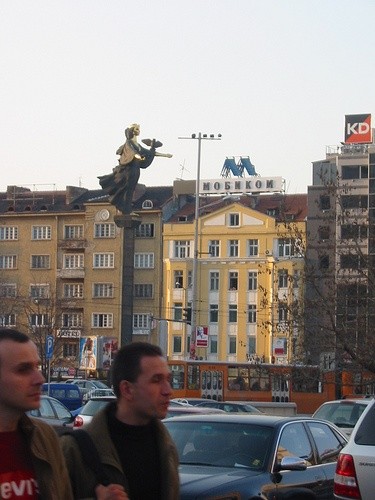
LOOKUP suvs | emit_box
[333,397,375,500]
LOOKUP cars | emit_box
[160,414,351,500]
[308,396,372,444]
[25,378,268,436]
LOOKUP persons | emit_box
[116,124,173,190]
[62,341,180,500]
[0,329,75,500]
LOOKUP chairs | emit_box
[231,434,267,467]
[183,436,222,465]
